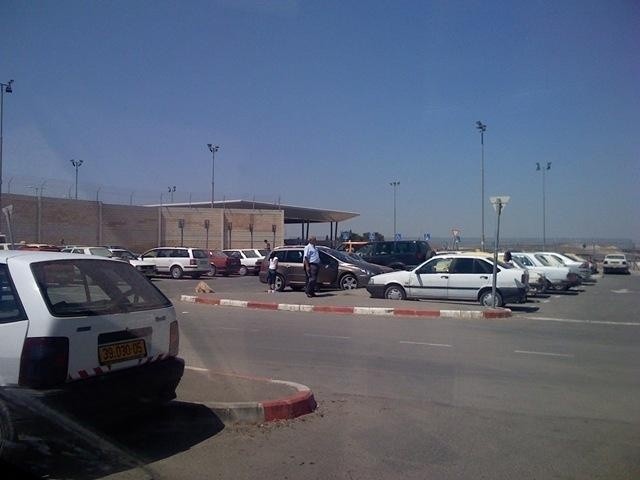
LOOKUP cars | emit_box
[602,251,629,275]
[0,236,268,283]
[366,249,599,309]
[0,253,183,459]
[342,251,363,261]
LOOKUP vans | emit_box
[349,240,436,273]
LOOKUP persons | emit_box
[303,236,320,298]
[266,251,279,293]
[503,251,512,262]
[263,239,270,249]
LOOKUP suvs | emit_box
[333,240,369,255]
[258,244,385,294]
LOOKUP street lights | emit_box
[474,117,490,248]
[389,180,401,242]
[69,157,86,201]
[206,142,222,210]
[534,158,553,251]
[1,77,14,232]
[167,183,178,209]
